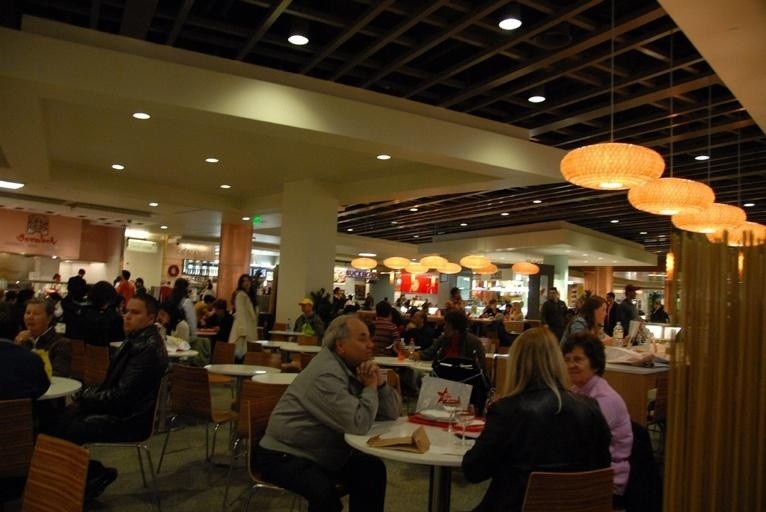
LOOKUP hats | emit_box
[298,297,314,306]
[173,277,189,290]
[625,284,640,293]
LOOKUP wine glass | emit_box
[442,394,475,448]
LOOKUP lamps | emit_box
[350,207,541,277]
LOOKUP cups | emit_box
[669,342,687,363]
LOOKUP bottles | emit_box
[636,333,666,354]
[286,318,292,332]
[613,321,624,348]
[187,260,217,276]
[398,338,414,360]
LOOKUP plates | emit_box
[419,408,451,421]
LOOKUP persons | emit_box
[364,293,434,351]
[255,315,401,512]
[445,287,466,315]
[295,287,356,336]
[160,275,258,362]
[541,285,667,347]
[480,299,523,320]
[462,328,632,511]
[410,312,487,376]
[1,269,169,502]
